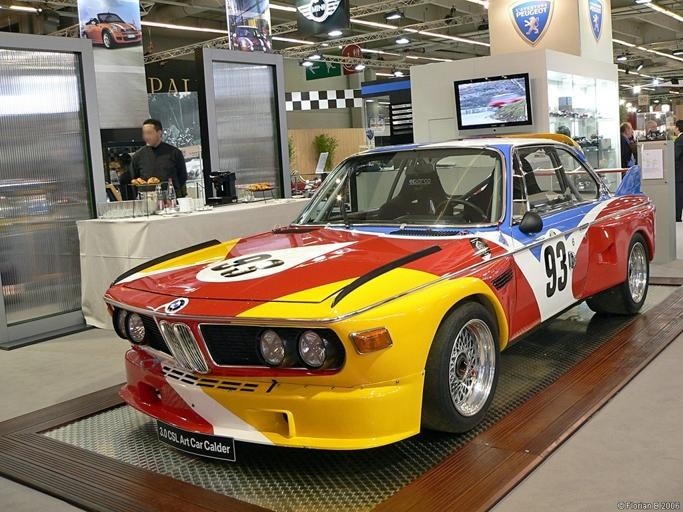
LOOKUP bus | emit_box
[0,177,54,220]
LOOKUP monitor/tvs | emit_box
[453,72,534,136]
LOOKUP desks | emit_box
[76,197,314,330]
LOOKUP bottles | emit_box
[166,177,177,209]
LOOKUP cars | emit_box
[487,92,523,111]
[95,128,657,466]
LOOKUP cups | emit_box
[96,199,148,219]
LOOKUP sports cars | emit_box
[230,24,270,52]
[80,9,141,50]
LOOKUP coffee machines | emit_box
[204,170,238,204]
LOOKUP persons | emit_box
[640,121,658,141]
[619,121,638,180]
[674,118,683,224]
[127,117,188,201]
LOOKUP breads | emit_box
[131,177,161,184]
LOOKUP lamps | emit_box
[302,9,412,78]
[618,51,662,112]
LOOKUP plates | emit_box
[127,183,163,186]
[245,187,275,192]
